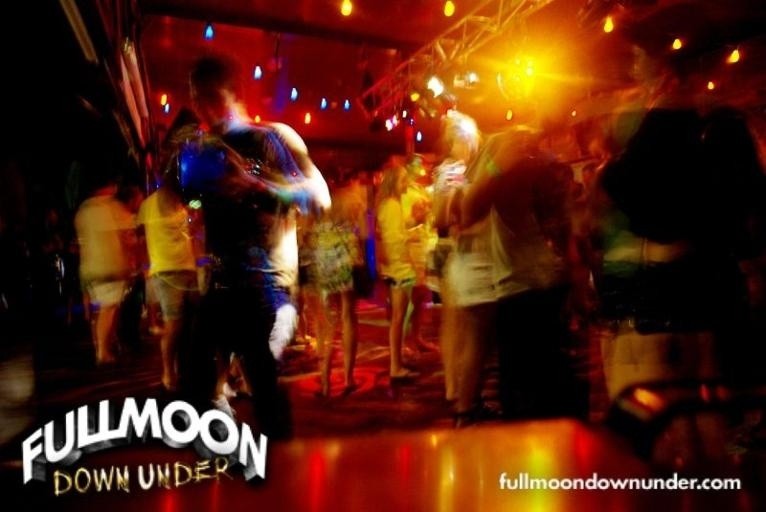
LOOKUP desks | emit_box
[0,416,704,512]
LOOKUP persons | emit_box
[76,9,766,512]
